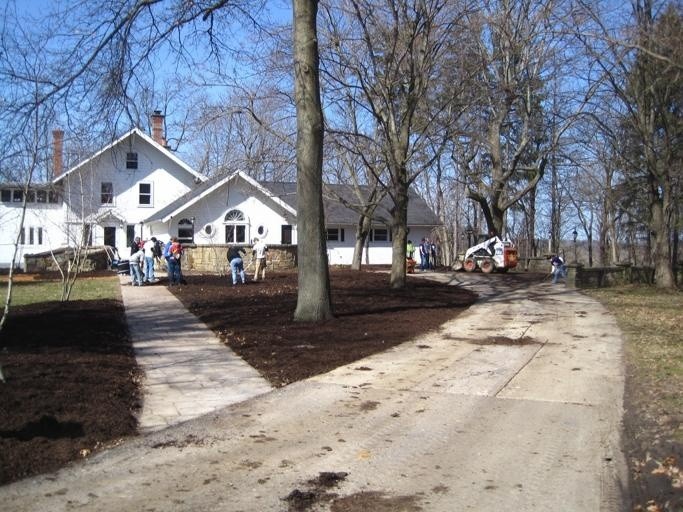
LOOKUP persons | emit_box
[557,247,567,276]
[406,237,436,272]
[250,237,269,283]
[225,243,248,287]
[546,254,567,284]
[127,235,184,287]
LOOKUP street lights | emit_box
[571,230,579,263]
[460,222,477,248]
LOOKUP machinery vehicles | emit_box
[451,232,519,273]
